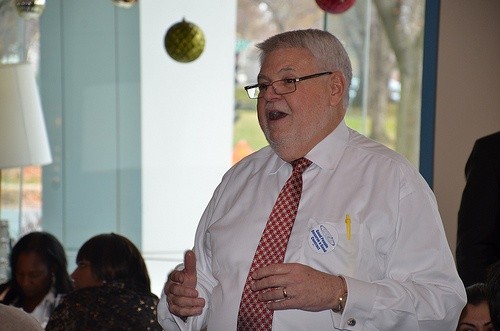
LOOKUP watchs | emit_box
[337,288,349,313]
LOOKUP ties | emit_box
[236,157,315,331]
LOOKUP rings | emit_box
[283,286,287,298]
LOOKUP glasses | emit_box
[244,71,333,98]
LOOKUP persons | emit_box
[0,230,74,331]
[456,283,495,331]
[156,28,469,331]
[454,131,500,331]
[44,232,166,331]
